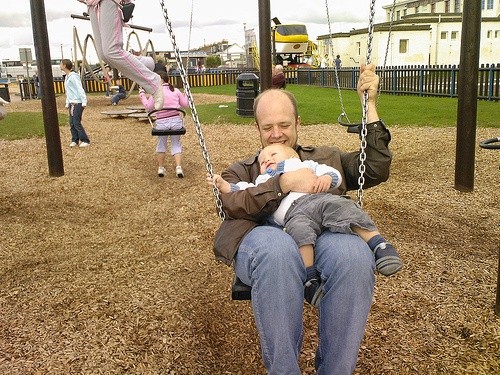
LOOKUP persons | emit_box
[60,58,91,148]
[78,0,166,110]
[138,71,191,178]
[206,142,402,308]
[212,63,393,375]
[30,54,342,107]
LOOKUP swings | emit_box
[159,0,376,307]
[325,0,397,133]
[123,0,193,137]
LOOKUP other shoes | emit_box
[373,241,403,276]
[303,277,325,308]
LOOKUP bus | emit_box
[1,59,66,78]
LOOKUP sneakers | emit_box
[175,166,185,178]
[79,142,90,148]
[69,142,77,146]
[157,166,168,177]
[153,75,165,110]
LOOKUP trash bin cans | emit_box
[236,73,259,116]
[0,83,10,102]
